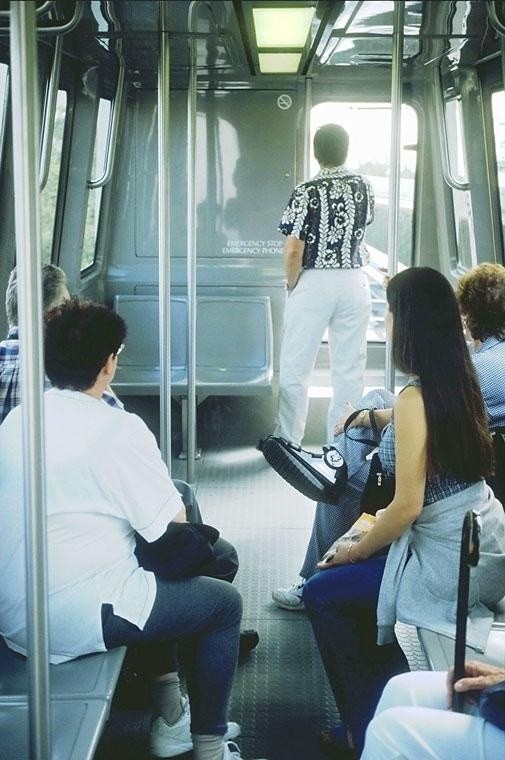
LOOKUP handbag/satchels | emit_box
[360,453,388,516]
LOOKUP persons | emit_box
[303,265,505,760]
[2,259,259,656]
[255,121,376,455]
[358,655,504,759]
[3,297,247,759]
[268,261,505,613]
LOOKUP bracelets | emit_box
[348,542,358,563]
[285,281,294,291]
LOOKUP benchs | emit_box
[0,480,195,758]
[104,295,274,460]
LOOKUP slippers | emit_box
[321,724,355,754]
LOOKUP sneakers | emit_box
[271,579,309,612]
[237,630,259,657]
[150,697,242,759]
[262,436,349,504]
